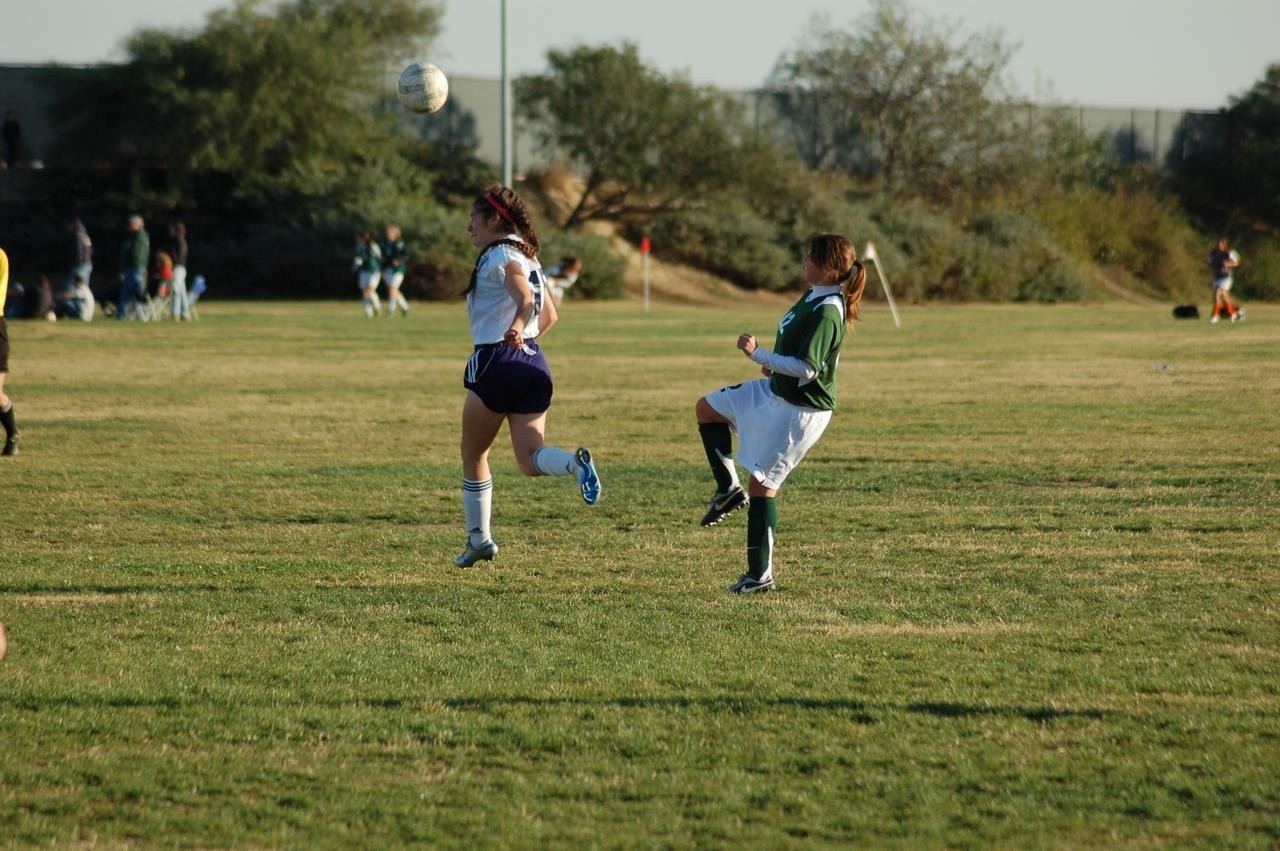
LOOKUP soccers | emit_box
[397,61,448,114]
[45,311,58,323]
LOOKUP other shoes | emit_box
[1209,314,1220,324]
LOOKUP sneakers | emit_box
[2,432,20,456]
[575,447,601,506]
[729,574,776,594]
[455,537,499,569]
[700,484,750,528]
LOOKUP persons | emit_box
[695,230,867,598]
[1206,238,1242,324]
[0,248,22,455]
[544,255,583,310]
[453,183,601,568]
[344,224,410,319]
[5,214,188,318]
